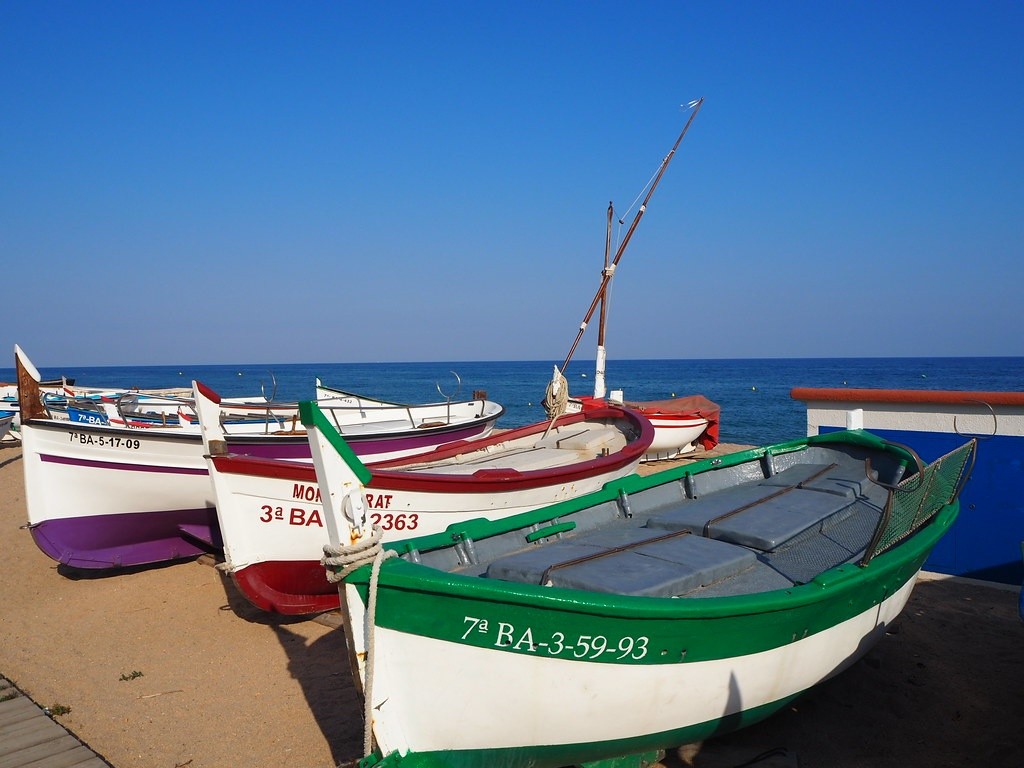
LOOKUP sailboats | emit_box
[545,99,719,465]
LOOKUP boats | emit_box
[192,379,653,619]
[10,343,504,576]
[296,399,976,767]
[1,378,300,449]
